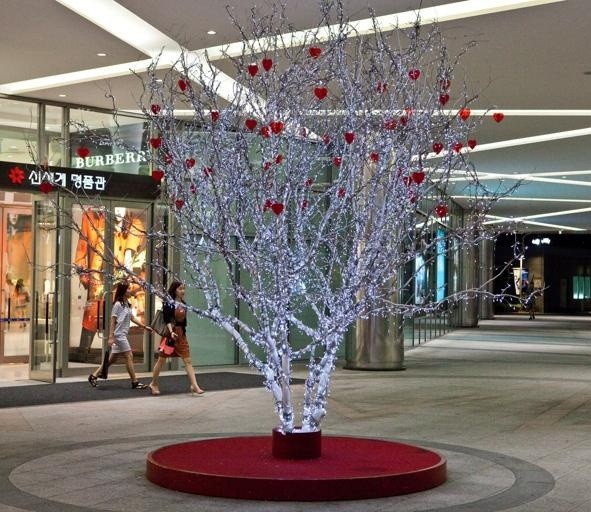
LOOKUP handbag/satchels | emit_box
[150,302,177,337]
[97,345,109,379]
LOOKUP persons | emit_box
[148,282,206,394]
[88,280,154,390]
[524,281,540,320]
[13,279,31,329]
[8,213,25,235]
[2,272,16,317]
[92,203,146,330]
[74,204,143,363]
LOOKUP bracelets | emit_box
[170,330,174,334]
[143,325,149,329]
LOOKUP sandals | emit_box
[131,381,148,389]
[87,374,98,387]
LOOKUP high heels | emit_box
[148,383,160,395]
[190,386,205,396]
